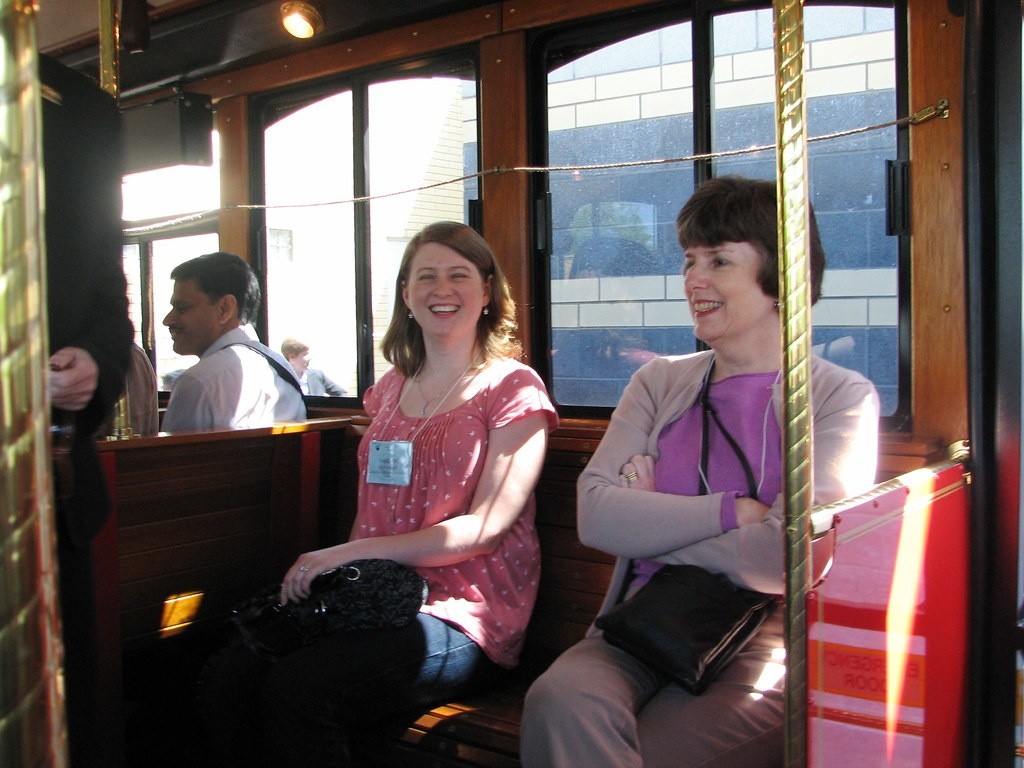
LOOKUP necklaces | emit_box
[418,381,445,415]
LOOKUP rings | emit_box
[626,471,638,484]
[301,567,307,572]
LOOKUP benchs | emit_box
[93,417,348,711]
[343,417,942,768]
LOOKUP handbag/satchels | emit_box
[223,558,429,648]
[594,562,776,693]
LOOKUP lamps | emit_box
[280,0,326,39]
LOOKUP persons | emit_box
[196,224,559,767]
[519,174,880,767]
[161,251,307,433]
[38,53,134,768]
[282,338,347,397]
